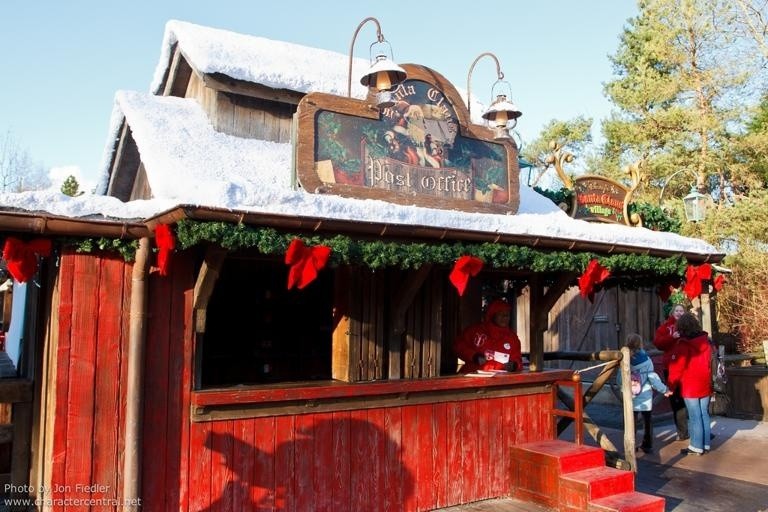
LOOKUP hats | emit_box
[396,101,423,119]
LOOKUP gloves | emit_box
[472,353,486,366]
[505,361,517,372]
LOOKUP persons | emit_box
[610,332,674,454]
[454,300,524,375]
[667,314,714,455]
[651,303,691,440]
[383,104,443,169]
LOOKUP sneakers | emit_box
[688,445,711,453]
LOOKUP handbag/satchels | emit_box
[709,352,731,415]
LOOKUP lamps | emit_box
[345,14,525,142]
[657,167,708,228]
